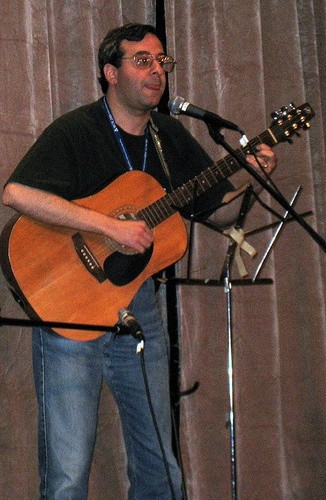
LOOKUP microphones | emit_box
[167,95,239,132]
[118,307,145,343]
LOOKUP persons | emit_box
[0,24,279,500]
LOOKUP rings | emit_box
[264,163,269,168]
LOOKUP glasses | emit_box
[121,53,177,73]
